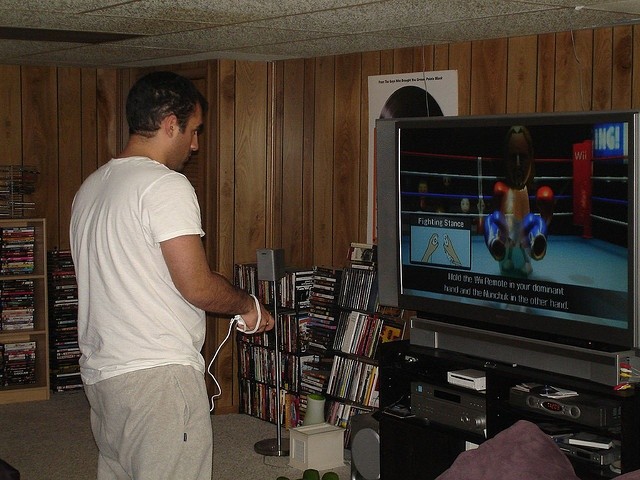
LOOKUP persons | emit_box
[69,69,275,479]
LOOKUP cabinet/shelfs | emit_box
[236,304,309,430]
[375,338,640,479]
[316,259,397,450]
[0,216,50,405]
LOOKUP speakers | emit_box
[352,412,381,479]
[256,248,287,282]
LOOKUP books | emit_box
[324,400,376,449]
[239,308,308,353]
[0,279,35,332]
[238,342,298,395]
[0,226,37,276]
[238,379,297,434]
[326,354,381,410]
[1,342,37,387]
[298,268,336,427]
[331,311,404,359]
[336,267,378,315]
[234,264,313,308]
[48,249,84,391]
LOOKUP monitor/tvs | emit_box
[375,110,639,388]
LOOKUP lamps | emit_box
[253,245,291,457]
